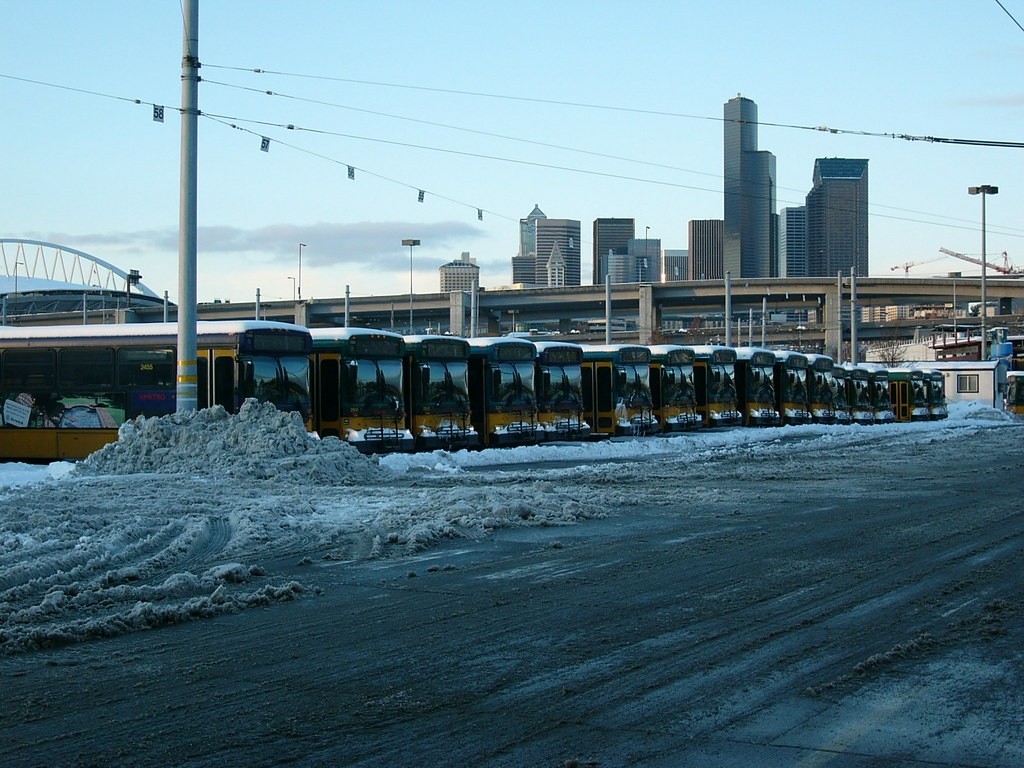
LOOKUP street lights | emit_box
[968,184,999,361]
[645,225,651,258]
[14,262,23,315]
[288,276,295,300]
[401,239,421,335]
[298,242,307,300]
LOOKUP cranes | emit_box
[890,255,947,277]
[939,247,1015,275]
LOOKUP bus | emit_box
[1005,371,1024,414]
[0,319,949,462]
